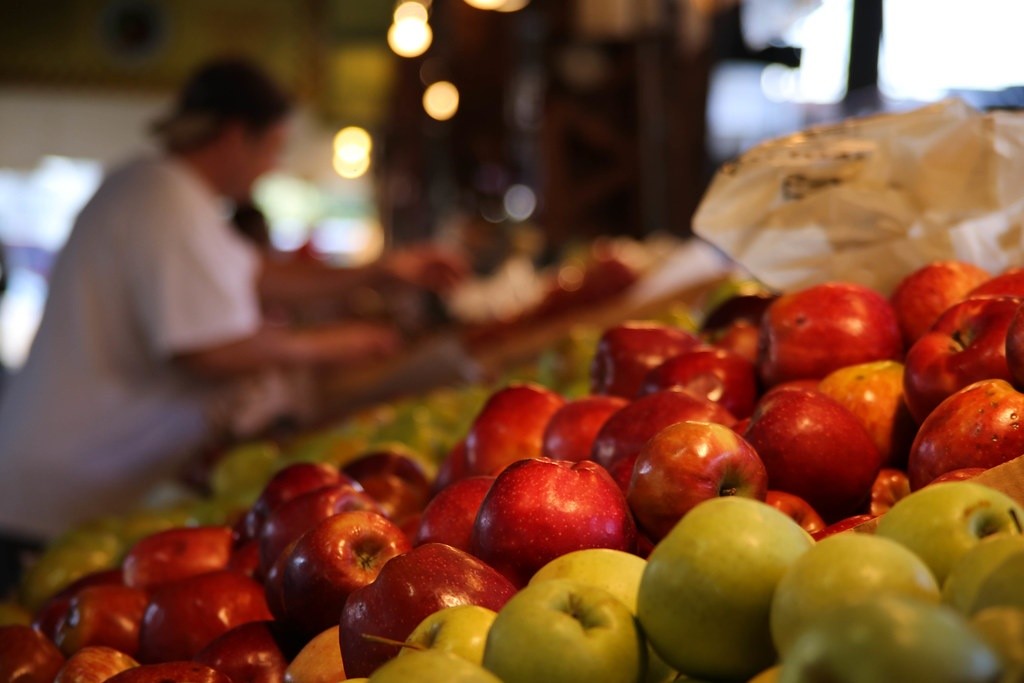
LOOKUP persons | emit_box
[0,51,405,602]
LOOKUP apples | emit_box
[0,259,1024,683]
